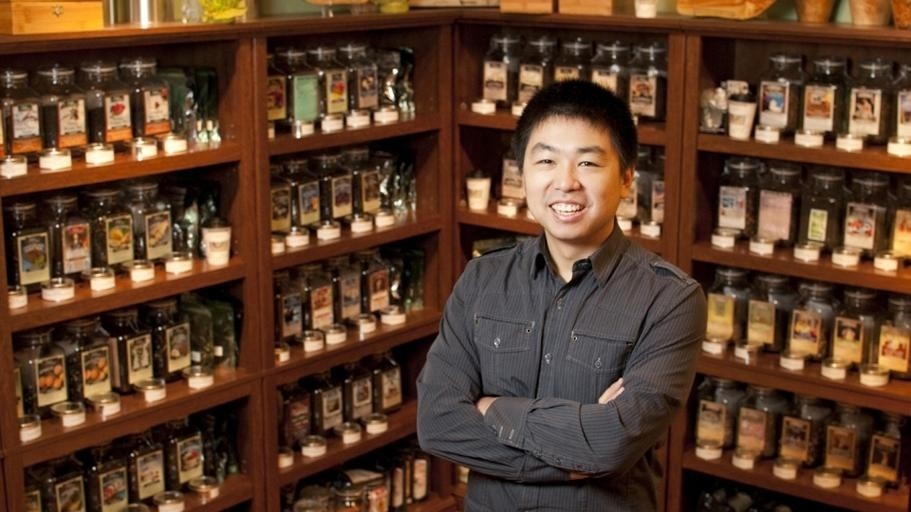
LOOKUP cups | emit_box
[464,169,492,211]
[792,0,911,29]
[632,0,660,19]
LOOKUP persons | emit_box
[417,77,710,511]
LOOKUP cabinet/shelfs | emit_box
[588,39,632,102]
[514,31,559,103]
[0,6,911,512]
[498,131,666,227]
[625,39,666,122]
[477,31,523,108]
[553,37,594,89]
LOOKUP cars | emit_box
[464,169,492,211]
[792,0,911,29]
[632,0,660,19]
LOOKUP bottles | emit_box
[588,39,632,102]
[477,31,523,108]
[625,39,666,122]
[689,48,910,510]
[514,31,559,103]
[498,131,666,227]
[267,32,435,511]
[553,37,594,89]
[0,53,235,510]
